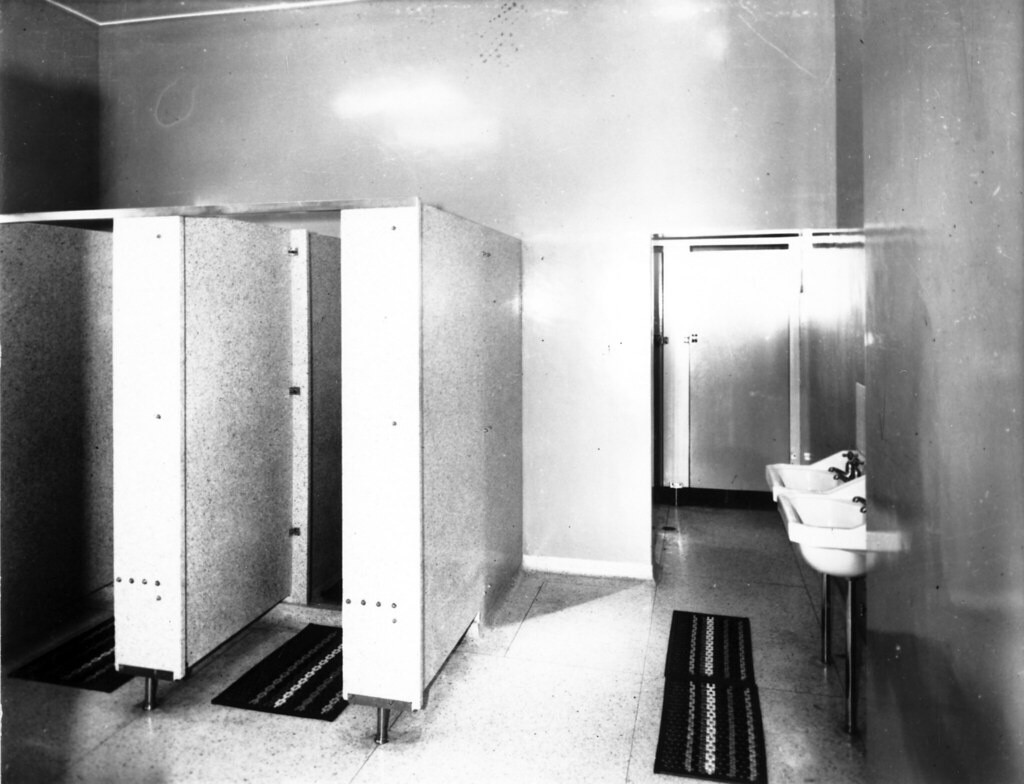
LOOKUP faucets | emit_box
[852,496,867,513]
[829,452,862,481]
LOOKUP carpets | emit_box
[655,610,768,784]
[6,618,135,696]
[208,622,349,724]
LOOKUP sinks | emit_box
[778,475,868,576]
[765,450,871,503]
